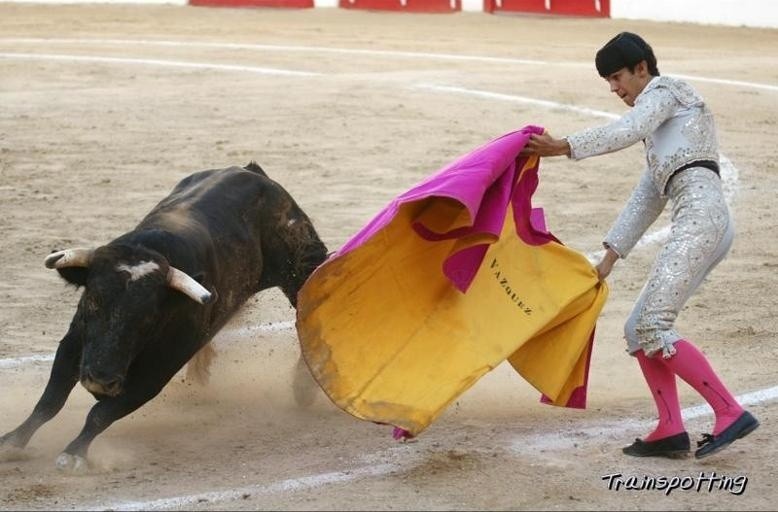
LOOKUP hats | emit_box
[595,32,650,79]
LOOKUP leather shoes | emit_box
[622,429,691,458]
[694,409,761,460]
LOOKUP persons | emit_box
[521,32,760,458]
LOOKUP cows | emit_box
[0,158,334,480]
